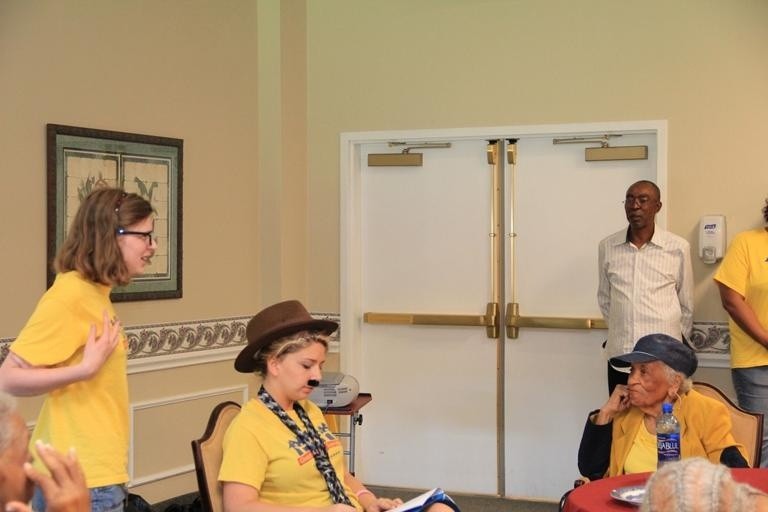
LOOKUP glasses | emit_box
[118,230,153,247]
[623,198,658,206]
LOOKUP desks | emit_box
[321,393,372,477]
[564,468,768,512]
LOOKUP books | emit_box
[385,487,461,512]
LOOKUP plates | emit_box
[610,486,648,506]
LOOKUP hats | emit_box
[235,300,338,373]
[639,457,768,512]
[610,335,697,377]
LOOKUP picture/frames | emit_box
[46,124,183,303]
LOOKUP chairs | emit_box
[693,382,765,468]
[192,402,242,512]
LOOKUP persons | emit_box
[1,188,160,512]
[0,391,91,512]
[597,181,694,402]
[713,198,768,467]
[216,299,456,512]
[578,334,752,482]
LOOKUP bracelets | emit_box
[356,489,374,497]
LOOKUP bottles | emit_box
[655,404,682,470]
